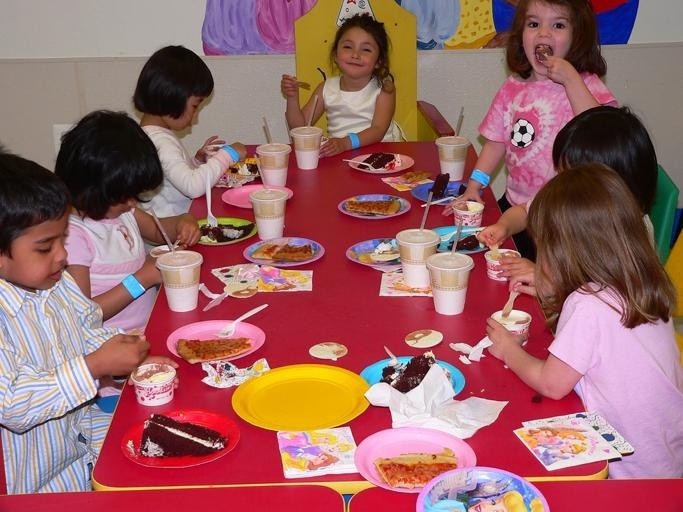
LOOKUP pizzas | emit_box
[251,244,312,263]
[345,199,404,216]
[176,338,252,364]
[374,447,458,489]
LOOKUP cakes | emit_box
[356,152,396,170]
[428,173,450,202]
[457,184,471,199]
[447,235,483,252]
[138,412,226,459]
[199,219,255,240]
[215,155,261,188]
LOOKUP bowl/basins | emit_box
[150,244,178,259]
[321,137,329,158]
[453,201,485,228]
[205,143,225,160]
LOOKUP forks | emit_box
[217,303,271,338]
[206,171,219,227]
[342,158,383,173]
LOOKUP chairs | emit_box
[291,1,455,143]
[647,163,679,266]
[662,231,683,367]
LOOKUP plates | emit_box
[121,411,242,469]
[349,152,414,174]
[222,185,294,208]
[416,467,551,512]
[243,237,325,267]
[360,357,466,410]
[412,181,482,207]
[166,319,266,363]
[231,363,372,433]
[338,194,412,220]
[425,225,496,255]
[187,218,257,246]
[346,237,405,264]
[354,427,477,493]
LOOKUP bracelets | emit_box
[469,169,491,190]
[121,274,146,301]
[223,143,240,164]
[346,132,360,150]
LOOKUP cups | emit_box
[434,136,470,182]
[156,250,203,312]
[250,188,288,239]
[290,126,323,169]
[427,252,475,315]
[255,142,292,187]
[396,228,440,289]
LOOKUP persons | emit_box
[281,10,404,158]
[54,108,202,399]
[131,45,247,251]
[0,142,151,495]
[485,162,683,480]
[440,1,618,217]
[476,107,658,251]
